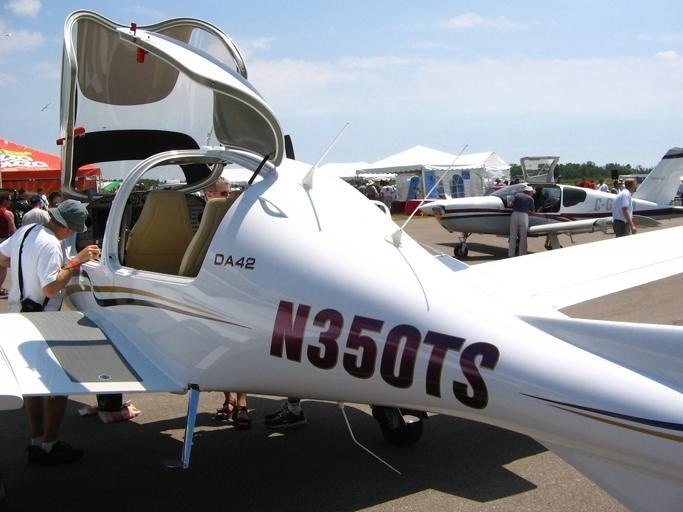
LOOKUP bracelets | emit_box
[70,258,79,271]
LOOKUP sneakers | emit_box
[266,406,307,428]
[28,440,84,466]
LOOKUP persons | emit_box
[493,178,520,191]
[199,176,252,432]
[508,186,537,258]
[0,199,100,467]
[356,178,397,213]
[264,397,307,430]
[0,187,63,297]
[611,179,637,238]
[576,177,626,194]
[679,180,683,206]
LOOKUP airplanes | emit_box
[0,5,682,512]
[413,144,682,260]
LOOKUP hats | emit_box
[48,200,89,233]
[523,186,536,193]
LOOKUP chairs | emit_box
[128,188,196,275]
[174,189,242,277]
[535,196,559,214]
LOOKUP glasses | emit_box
[212,191,228,196]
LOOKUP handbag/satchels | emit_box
[20,299,43,312]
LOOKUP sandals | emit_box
[216,399,251,430]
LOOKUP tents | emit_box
[423,151,511,200]
[0,138,100,195]
